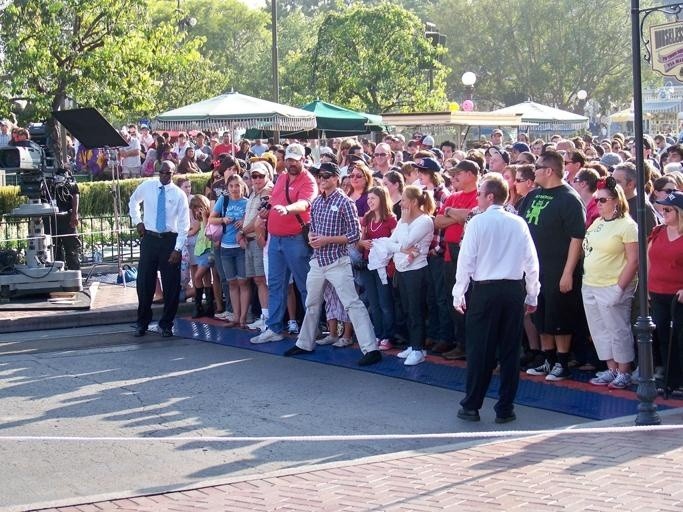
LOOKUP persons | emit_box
[42,175,81,270]
[451,170,544,425]
[66,133,123,181]
[0,120,30,186]
[117,118,683,400]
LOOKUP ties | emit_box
[155,186,166,231]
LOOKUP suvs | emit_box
[28,122,49,153]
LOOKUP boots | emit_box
[191,286,204,318]
[205,286,214,317]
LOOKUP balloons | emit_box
[446,99,476,114]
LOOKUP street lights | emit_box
[417,31,446,93]
[462,70,476,100]
[576,89,586,115]
[664,80,674,94]
[179,14,196,40]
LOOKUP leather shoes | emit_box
[162,326,172,337]
[495,411,516,423]
[457,407,480,421]
[135,326,148,336]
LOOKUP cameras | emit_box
[257,201,272,211]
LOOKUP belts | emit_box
[146,231,175,239]
[246,237,255,241]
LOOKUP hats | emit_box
[320,163,340,174]
[251,163,266,174]
[493,129,502,138]
[347,153,369,166]
[285,144,304,161]
[656,191,683,207]
[411,158,440,171]
[448,160,480,174]
[488,143,536,164]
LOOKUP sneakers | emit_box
[288,320,299,334]
[379,338,467,365]
[315,336,353,347]
[359,351,382,366]
[285,346,312,356]
[590,366,683,399]
[519,349,572,381]
[214,311,284,343]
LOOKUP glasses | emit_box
[595,198,611,203]
[374,153,387,156]
[317,172,331,179]
[350,174,362,178]
[252,175,264,178]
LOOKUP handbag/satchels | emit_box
[302,223,314,249]
[118,264,137,283]
[205,222,224,240]
